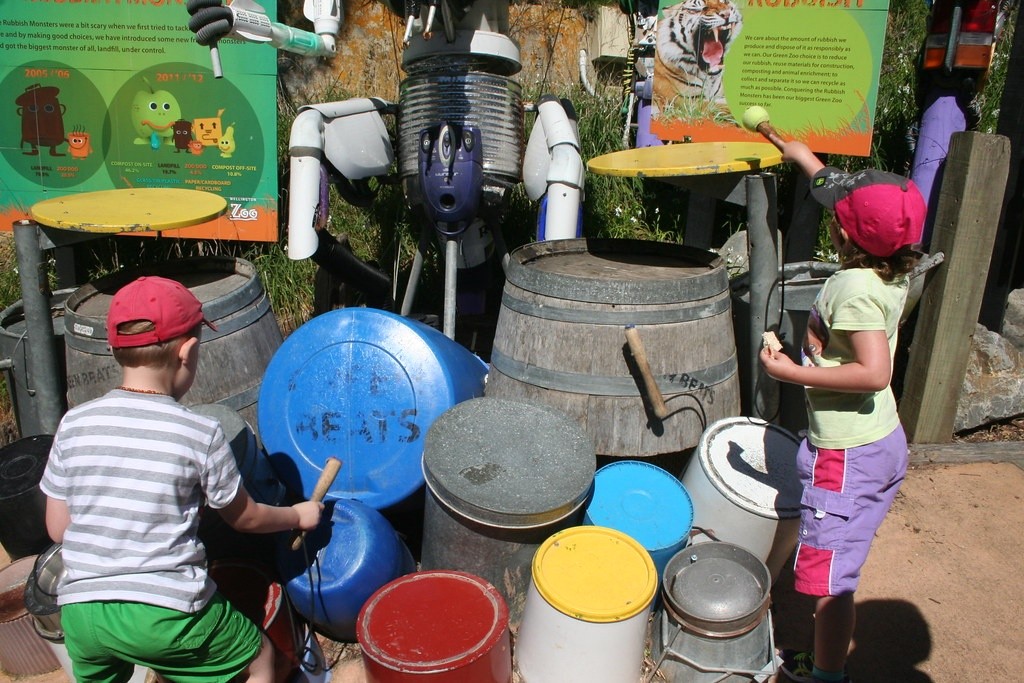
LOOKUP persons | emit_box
[760,140,926,683]
[39,276,325,683]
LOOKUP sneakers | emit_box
[779,649,851,683]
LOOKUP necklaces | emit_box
[115,386,169,395]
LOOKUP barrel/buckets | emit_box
[0,288,84,437]
[730,261,844,437]
[514,525,658,683]
[678,416,802,586]
[63,255,286,452]
[257,306,489,509]
[420,397,595,650]
[0,434,55,561]
[486,239,741,456]
[23,544,151,683]
[581,461,695,613]
[275,499,416,643]
[356,570,513,683]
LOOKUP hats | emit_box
[810,165,928,259]
[107,275,218,349]
